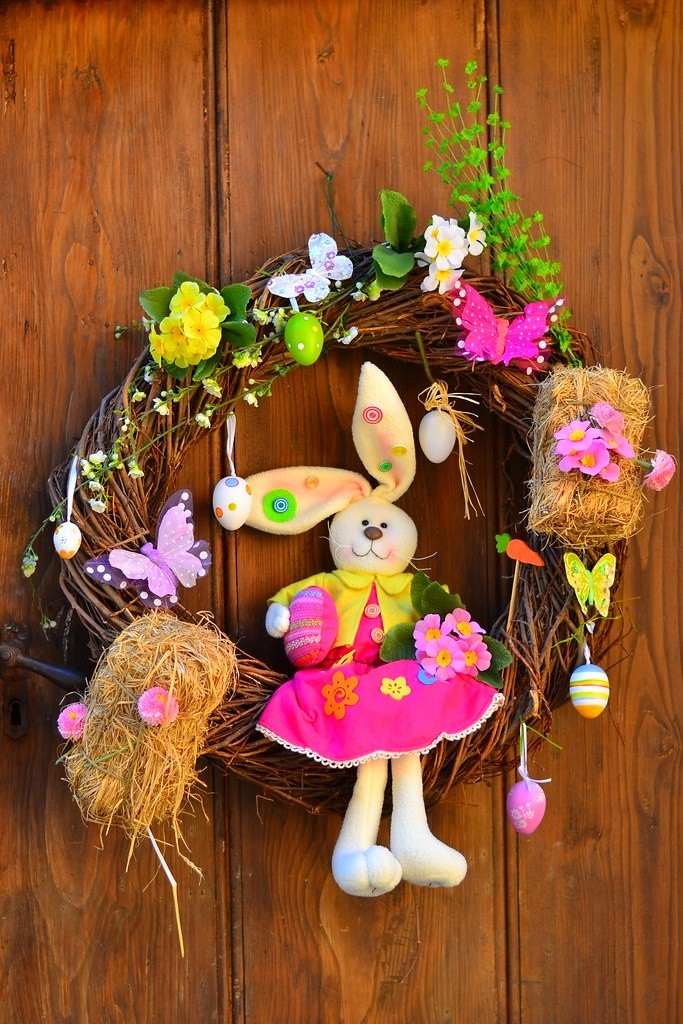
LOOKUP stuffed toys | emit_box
[240,362,505,898]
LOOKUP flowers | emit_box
[137,688,181,728]
[553,401,676,492]
[19,53,587,638]
[56,702,89,740]
[378,570,513,689]
